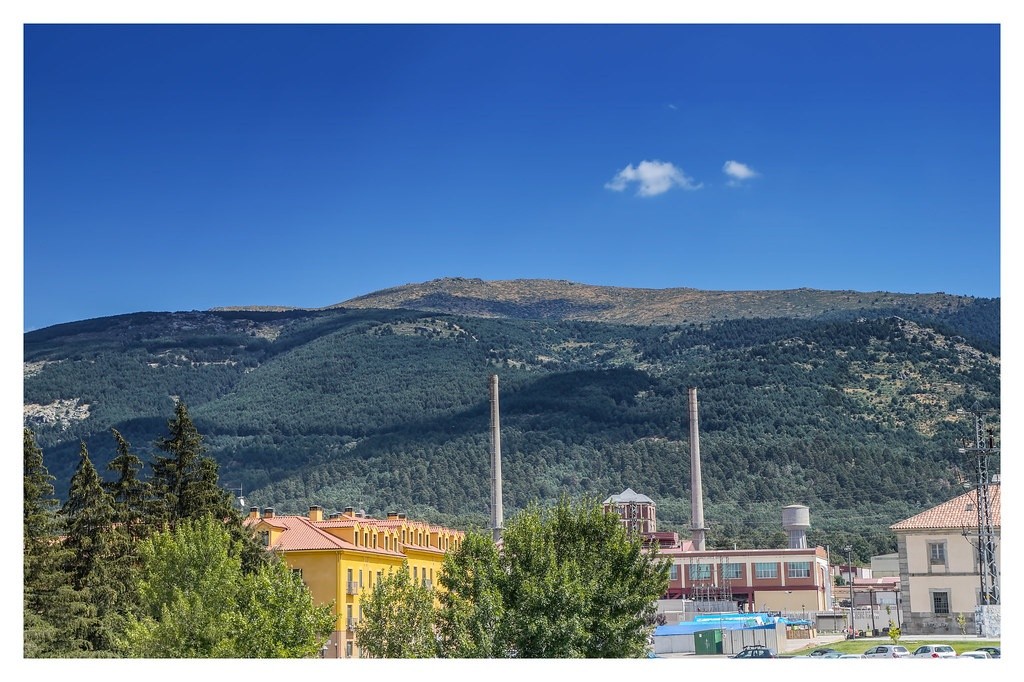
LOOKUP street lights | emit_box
[893,586,901,628]
[868,585,876,637]
[843,548,856,640]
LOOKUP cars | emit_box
[975,647,1001,659]
[807,648,866,659]
[960,651,992,659]
[730,648,778,658]
[911,644,957,658]
[862,645,911,659]
